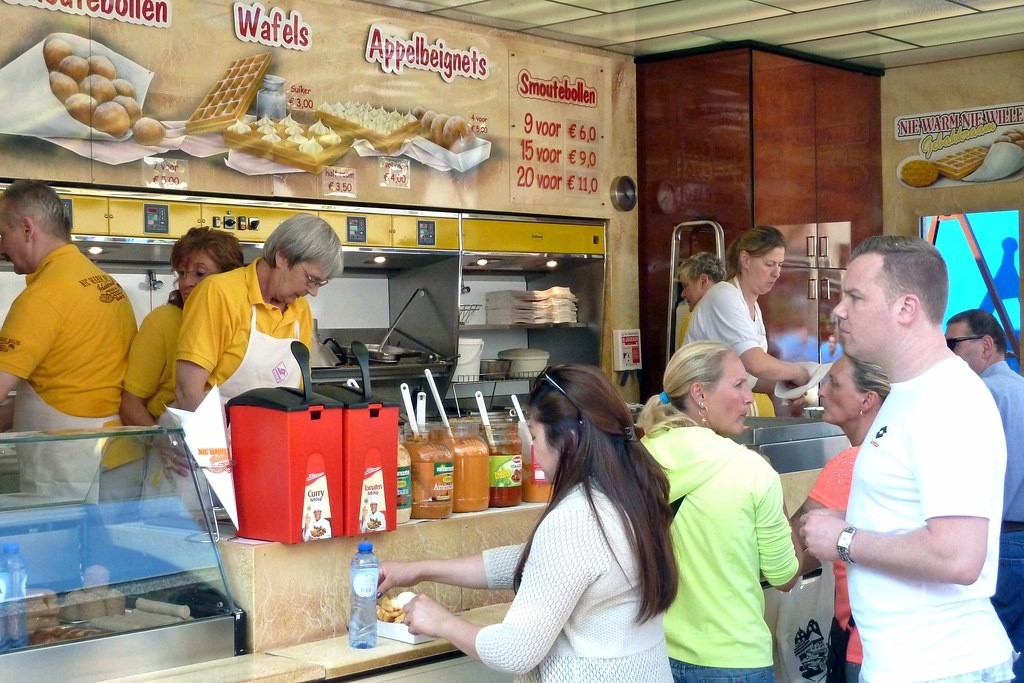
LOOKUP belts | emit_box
[1001,521,1024,531]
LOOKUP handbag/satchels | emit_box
[775,551,836,683]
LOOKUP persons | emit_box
[944,310,1024,683]
[377,364,679,683]
[0,181,138,504]
[680,226,810,417]
[142,214,344,512]
[800,236,1016,683]
[677,252,728,313]
[637,340,803,683]
[823,335,842,363]
[789,353,891,683]
[98,226,244,503]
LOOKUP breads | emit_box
[182,52,272,135]
[901,145,989,186]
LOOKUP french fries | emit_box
[376,596,406,625]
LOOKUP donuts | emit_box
[410,105,477,154]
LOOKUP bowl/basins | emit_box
[343,343,422,367]
[497,348,550,377]
[480,360,511,379]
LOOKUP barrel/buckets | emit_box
[450,338,484,382]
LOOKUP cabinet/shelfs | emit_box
[635,37,888,392]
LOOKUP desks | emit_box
[154,598,516,682]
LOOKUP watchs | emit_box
[837,526,857,564]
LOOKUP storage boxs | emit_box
[374,618,438,645]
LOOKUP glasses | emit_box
[301,259,328,287]
[170,268,226,279]
[533,365,583,425]
[946,336,995,351]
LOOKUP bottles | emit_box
[256,74,285,120]
[397,420,555,524]
[0,543,28,650]
[348,543,379,647]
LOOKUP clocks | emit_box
[610,175,637,212]
[653,179,678,214]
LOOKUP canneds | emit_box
[397,409,557,523]
